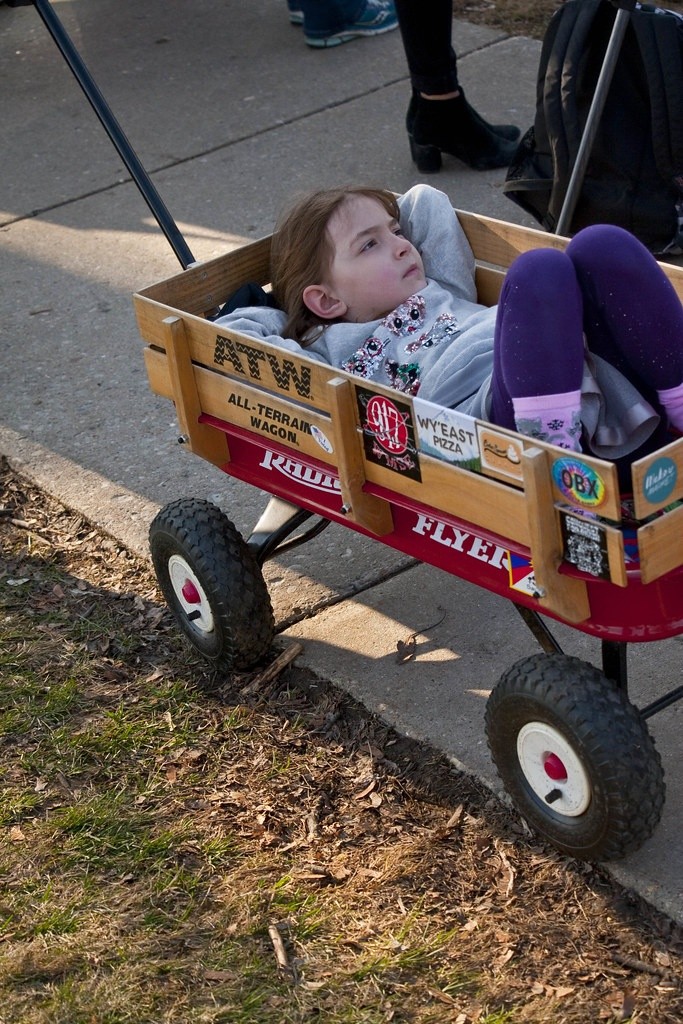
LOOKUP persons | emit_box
[393,0,521,175]
[287,0,399,48]
[207,183,683,521]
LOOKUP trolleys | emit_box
[29,0,683,861]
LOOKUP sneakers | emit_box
[289,0,399,47]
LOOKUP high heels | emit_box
[406,85,521,173]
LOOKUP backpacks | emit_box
[502,0,683,260]
